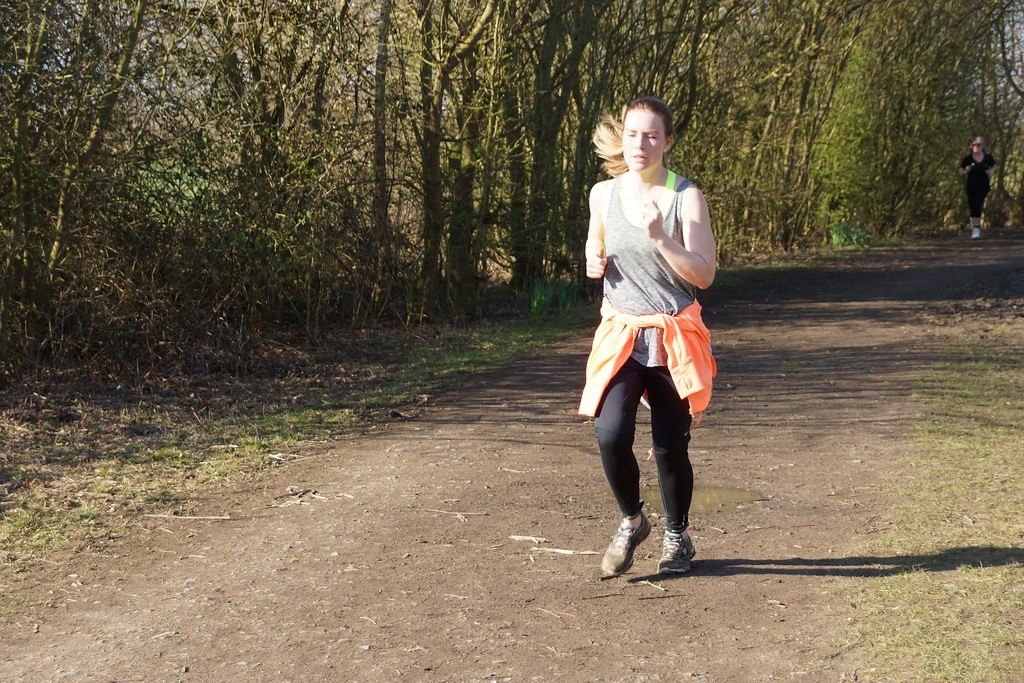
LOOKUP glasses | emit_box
[972,144,981,146]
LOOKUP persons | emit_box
[959,137,1000,239]
[584,96,716,575]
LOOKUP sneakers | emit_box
[657,529,695,574]
[601,511,651,575]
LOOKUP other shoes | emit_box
[972,228,979,239]
